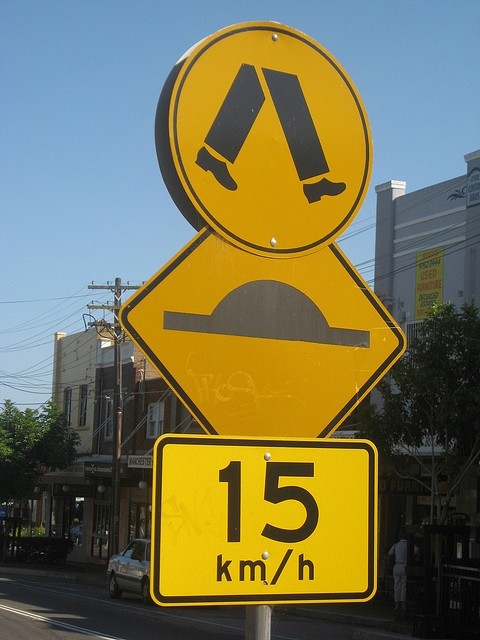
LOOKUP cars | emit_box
[106,538,156,605]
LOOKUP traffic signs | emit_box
[147,432,381,608]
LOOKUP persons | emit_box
[388,527,420,611]
[70,517,81,543]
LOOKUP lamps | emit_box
[33,481,40,494]
[98,477,106,493]
[138,468,148,490]
[62,470,70,492]
